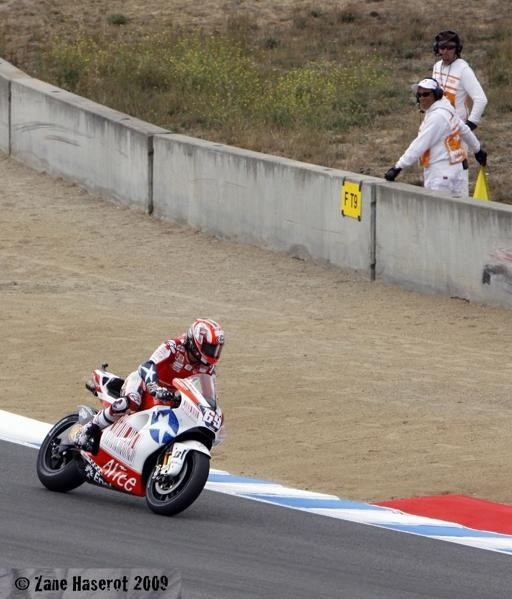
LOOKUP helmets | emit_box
[186,318,223,366]
[431,30,463,59]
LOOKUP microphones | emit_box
[418,102,424,114]
[435,53,442,56]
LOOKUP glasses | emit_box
[437,44,457,51]
[416,90,431,98]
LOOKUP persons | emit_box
[384,31,488,197]
[74,319,224,452]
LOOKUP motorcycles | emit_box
[37,363,224,516]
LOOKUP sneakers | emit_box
[73,423,102,454]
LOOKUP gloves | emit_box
[384,166,401,183]
[465,121,476,130]
[474,150,488,166]
[153,386,174,401]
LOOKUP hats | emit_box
[410,79,439,94]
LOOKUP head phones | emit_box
[416,77,443,103]
[433,31,462,54]
